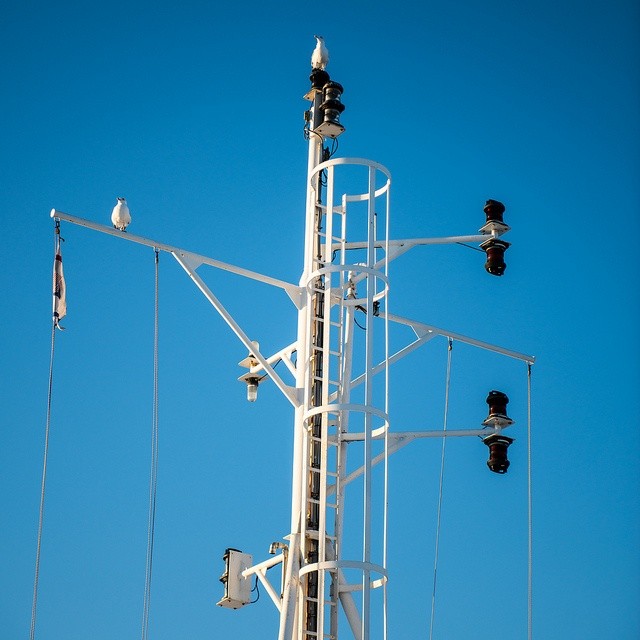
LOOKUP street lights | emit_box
[240,341,296,403]
[350,391,515,475]
[219,548,284,610]
[342,199,510,277]
